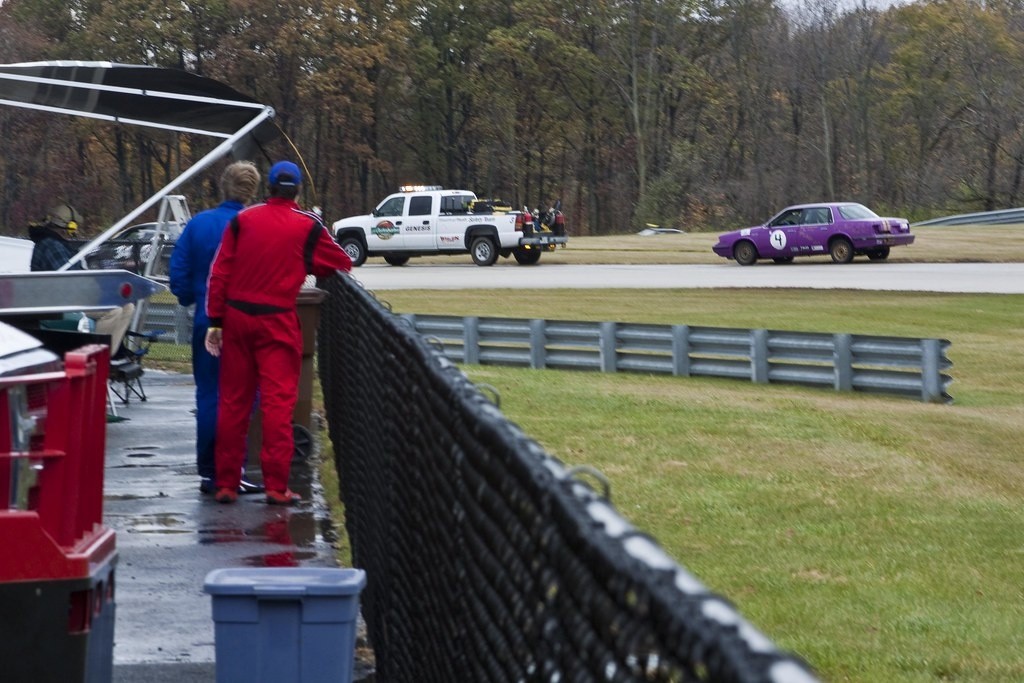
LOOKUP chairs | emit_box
[106,332,158,403]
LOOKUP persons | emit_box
[28,204,142,362]
[203,161,352,505]
[168,164,268,496]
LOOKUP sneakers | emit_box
[215,489,239,502]
[266,488,302,506]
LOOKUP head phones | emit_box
[66,205,79,235]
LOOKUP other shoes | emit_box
[108,357,132,366]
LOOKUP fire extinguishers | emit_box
[555,209,564,237]
[523,205,532,238]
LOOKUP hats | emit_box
[268,161,301,186]
[47,206,84,226]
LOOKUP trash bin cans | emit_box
[203,567,368,683]
[240,287,331,468]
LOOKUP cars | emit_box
[712,202,915,265]
[636,227,683,237]
[111,221,180,275]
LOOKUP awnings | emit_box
[0,56,317,280]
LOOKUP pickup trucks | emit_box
[333,184,567,267]
